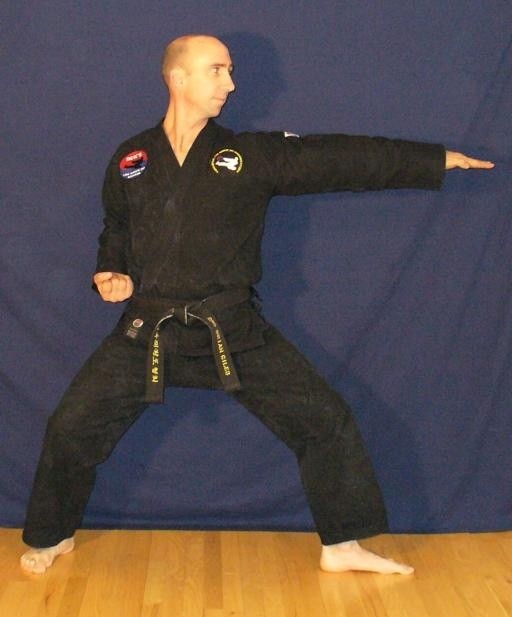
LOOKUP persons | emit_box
[19,33,495,577]
